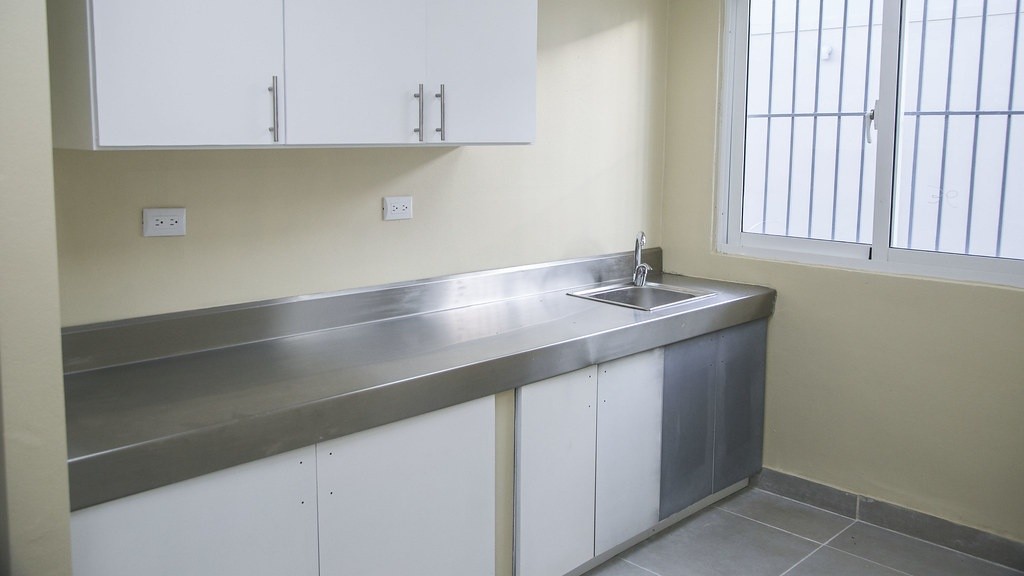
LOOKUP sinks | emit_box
[566,277,719,312]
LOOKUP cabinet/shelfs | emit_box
[284,1,538,148]
[71,393,495,576]
[47,0,286,151]
[515,346,664,576]
[659,317,767,524]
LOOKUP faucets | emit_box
[633,231,652,287]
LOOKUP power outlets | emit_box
[383,196,413,221]
[143,208,186,238]
